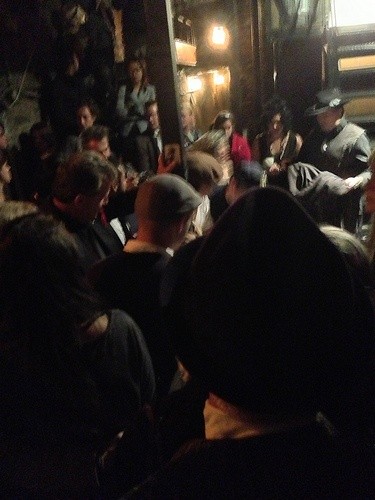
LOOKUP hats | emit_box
[158,185,375,411]
[304,87,352,115]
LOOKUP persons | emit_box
[0,54,374,499]
